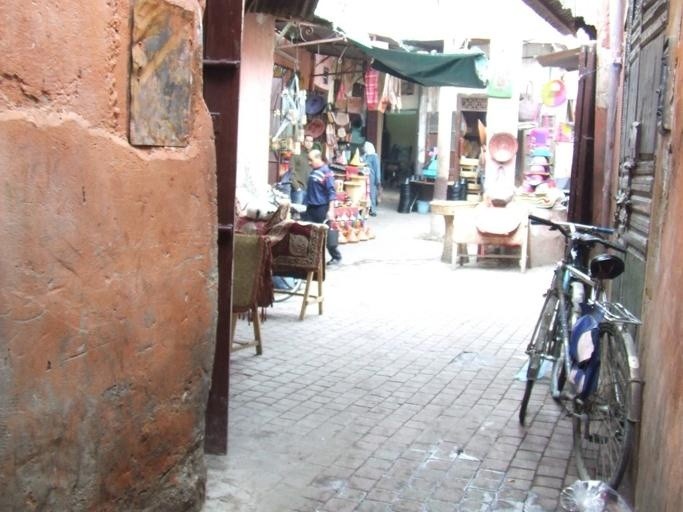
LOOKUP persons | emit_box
[360,140,380,215]
[290,135,314,219]
[306,149,342,265]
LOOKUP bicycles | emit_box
[518,214,648,494]
